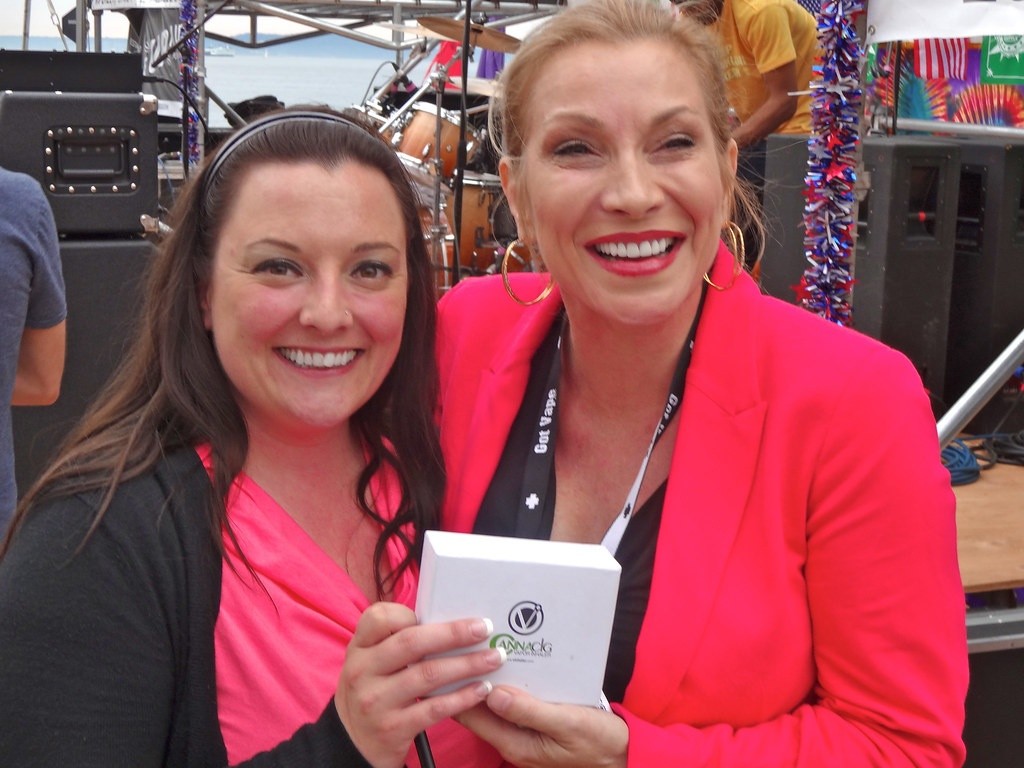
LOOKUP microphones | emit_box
[391,63,419,97]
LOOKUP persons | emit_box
[0,105,508,768]
[400,0,968,768]
[679,0,819,151]
[0,166,66,543]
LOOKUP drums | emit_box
[307,100,548,285]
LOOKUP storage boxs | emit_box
[412,529,621,707]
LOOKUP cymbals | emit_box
[416,15,522,54]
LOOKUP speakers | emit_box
[757,133,1024,436]
[0,86,163,513]
